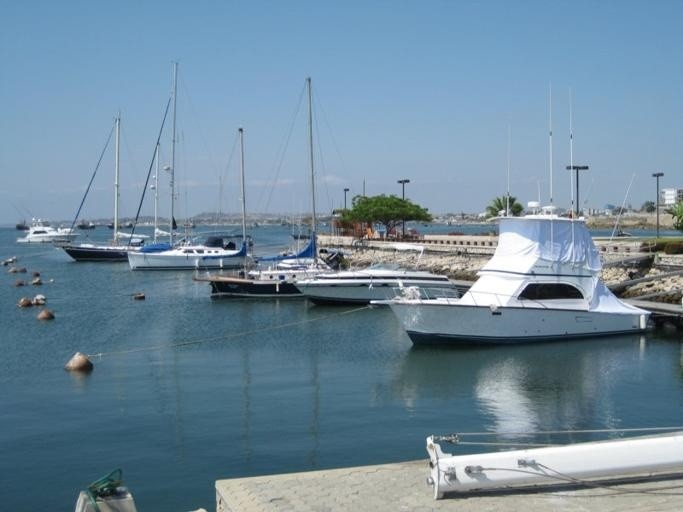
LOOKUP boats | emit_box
[9,219,139,247]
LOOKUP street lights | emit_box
[650,171,664,239]
